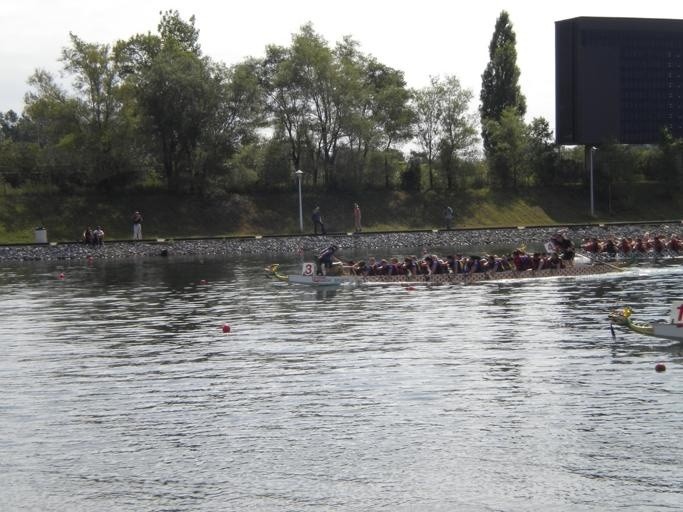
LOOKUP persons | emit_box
[310,204,327,234]
[81,224,96,249]
[94,226,105,248]
[443,205,453,229]
[351,202,362,232]
[131,210,142,239]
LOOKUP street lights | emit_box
[295,170,304,233]
[589,146,598,217]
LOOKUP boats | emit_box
[575,250,683,261]
[608,306,683,340]
[271,253,624,286]
